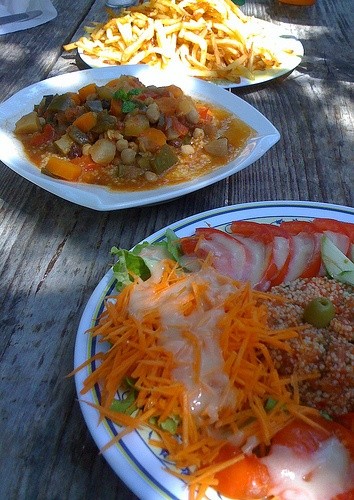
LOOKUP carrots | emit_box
[67,267,354,500]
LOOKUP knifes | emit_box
[0,9,42,26]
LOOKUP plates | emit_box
[76,14,304,89]
[0,65,282,212]
[75,200,354,500]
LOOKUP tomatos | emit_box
[178,219,354,294]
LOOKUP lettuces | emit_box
[109,227,188,289]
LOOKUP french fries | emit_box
[64,0,295,83]
